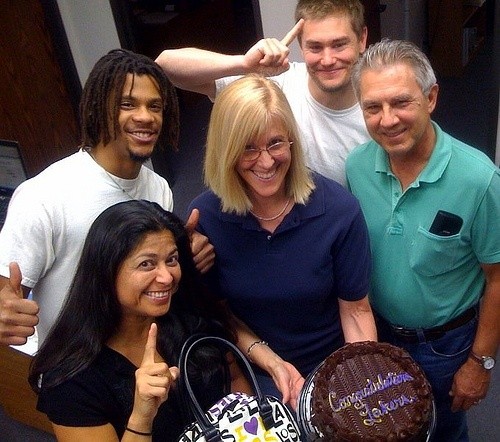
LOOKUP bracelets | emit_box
[126,427,152,436]
[247,340,268,363]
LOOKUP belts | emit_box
[375,304,480,341]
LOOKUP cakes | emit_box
[300,341,433,442]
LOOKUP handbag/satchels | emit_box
[177,330,305,442]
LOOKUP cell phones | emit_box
[429,210,464,237]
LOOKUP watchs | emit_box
[469,351,495,370]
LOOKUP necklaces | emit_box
[248,198,290,220]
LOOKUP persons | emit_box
[28,200,253,442]
[345,39,500,442]
[180,74,378,413]
[0,48,214,349]
[154,0,373,189]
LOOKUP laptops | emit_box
[0,138,26,229]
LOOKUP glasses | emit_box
[239,139,293,161]
